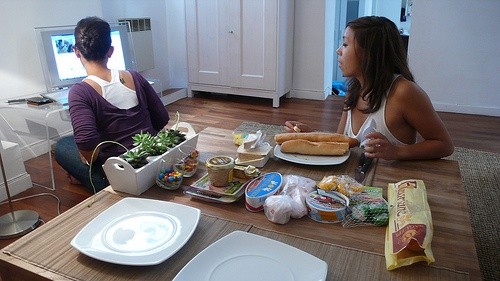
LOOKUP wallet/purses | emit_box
[26,96,53,106]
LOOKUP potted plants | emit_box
[100,121,199,196]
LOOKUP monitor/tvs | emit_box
[34,20,138,93]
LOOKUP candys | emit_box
[159,168,182,188]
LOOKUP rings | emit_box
[375,143,381,148]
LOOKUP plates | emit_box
[70,197,201,266]
[274,144,350,165]
[172,230,328,281]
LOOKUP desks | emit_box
[0,89,70,191]
[0,126,482,281]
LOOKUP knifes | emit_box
[355,152,373,183]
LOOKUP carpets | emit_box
[234,120,500,281]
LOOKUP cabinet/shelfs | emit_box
[184,0,293,109]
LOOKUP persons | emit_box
[55,39,64,53]
[54,15,170,191]
[284,15,454,161]
[64,40,74,52]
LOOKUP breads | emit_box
[274,126,357,155]
[406,239,424,254]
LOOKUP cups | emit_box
[173,146,200,177]
[205,155,235,187]
[155,156,186,190]
